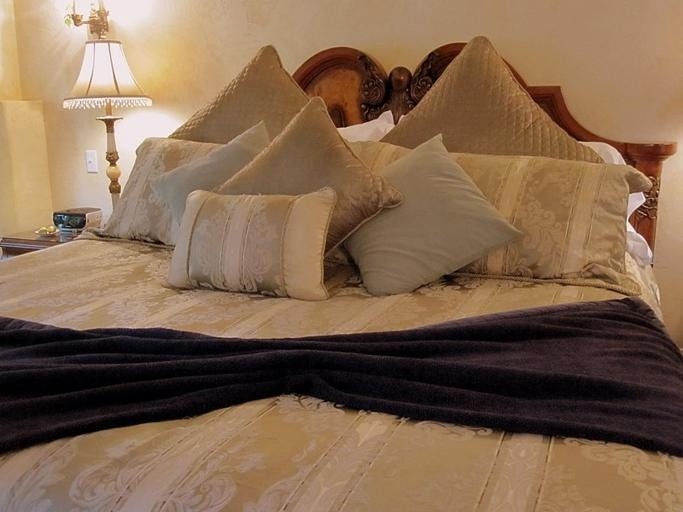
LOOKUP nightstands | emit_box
[0,225,73,260]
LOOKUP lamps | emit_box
[64,37,152,216]
[64,0,110,37]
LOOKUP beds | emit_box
[0,44,683,511]
